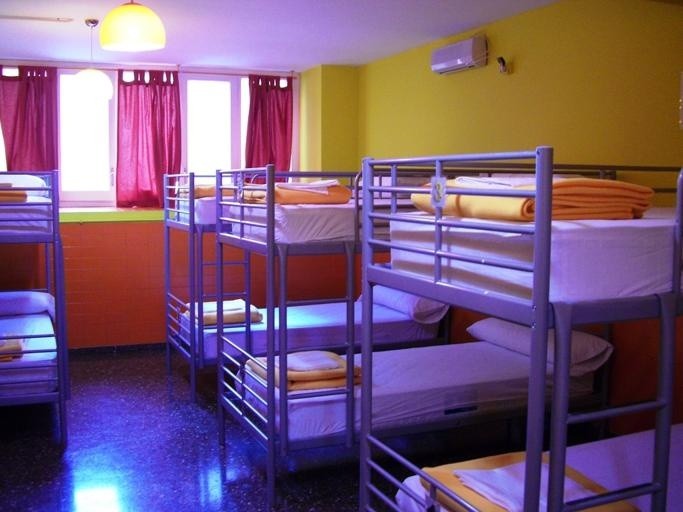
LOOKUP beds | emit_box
[0,169,71,443]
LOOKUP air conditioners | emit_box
[428,35,488,75]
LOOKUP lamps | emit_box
[99,0,166,55]
[72,19,113,102]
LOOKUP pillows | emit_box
[0,292,56,322]
[1,175,49,198]
